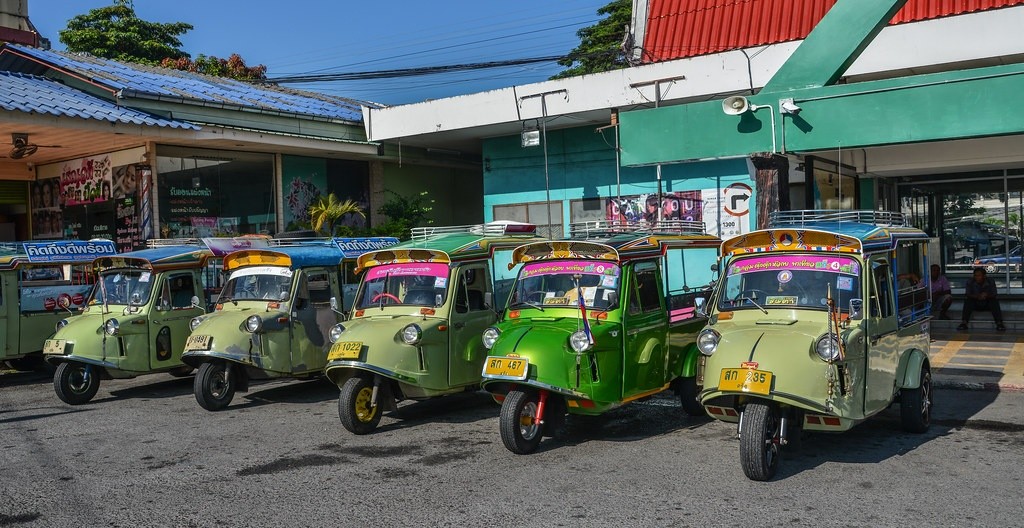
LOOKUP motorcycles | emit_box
[0,209,936,482]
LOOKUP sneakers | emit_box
[939,315,950,320]
[996,324,1006,331]
[957,323,968,330]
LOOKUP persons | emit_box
[896,271,920,293]
[957,267,1007,332]
[102,183,110,200]
[662,194,681,220]
[122,165,137,197]
[917,264,953,320]
[53,178,62,207]
[32,211,62,236]
[32,181,41,208]
[645,195,659,224]
[61,186,81,204]
[40,179,54,207]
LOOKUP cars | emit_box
[970,244,1022,279]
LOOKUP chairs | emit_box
[258,281,285,304]
[402,290,442,307]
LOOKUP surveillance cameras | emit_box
[778,98,802,116]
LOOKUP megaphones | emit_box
[721,96,750,115]
